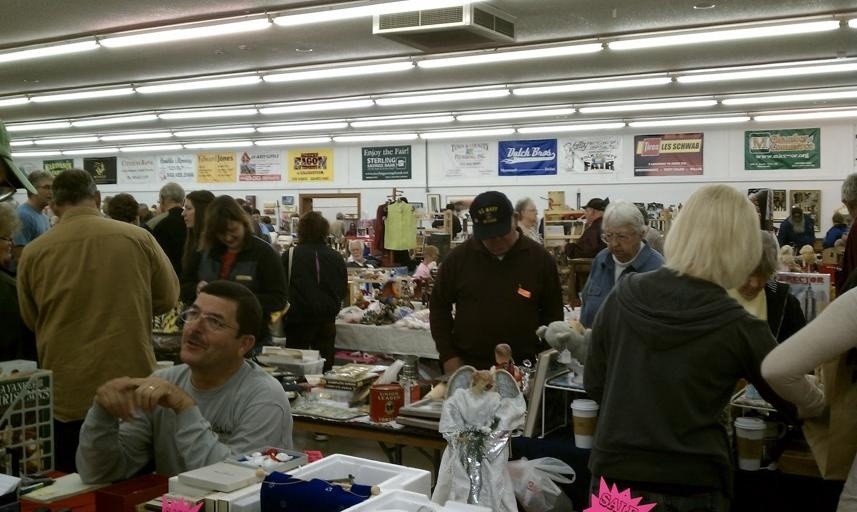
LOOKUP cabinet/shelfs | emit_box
[544,210,587,246]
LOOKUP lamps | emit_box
[578,99,719,115]
[676,62,857,86]
[6,123,72,132]
[136,76,263,94]
[100,17,272,49]
[513,78,673,96]
[722,91,849,105]
[99,133,173,141]
[0,95,29,108]
[262,61,414,83]
[518,123,626,134]
[173,127,256,137]
[10,141,34,146]
[417,42,603,70]
[259,100,374,116]
[62,149,119,155]
[376,88,511,108]
[456,109,576,123]
[184,141,253,148]
[0,39,99,64]
[34,137,98,144]
[72,114,158,128]
[607,20,841,51]
[350,115,454,128]
[754,112,850,122]
[120,145,183,153]
[10,151,61,157]
[254,136,331,147]
[333,134,419,143]
[420,127,516,141]
[272,5,375,27]
[257,122,349,133]
[29,88,134,104]
[627,116,751,128]
[159,110,257,120]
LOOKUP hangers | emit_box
[383,194,415,213]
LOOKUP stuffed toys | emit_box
[795,245,819,273]
[777,245,793,272]
[354,279,415,326]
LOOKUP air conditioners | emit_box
[373,2,517,52]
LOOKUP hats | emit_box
[468,190,514,241]
[580,198,610,211]
[0,119,39,195]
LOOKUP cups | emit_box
[570,399,599,449]
[735,416,768,471]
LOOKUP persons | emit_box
[280,210,348,373]
[432,204,462,240]
[243,206,271,245]
[141,183,186,274]
[637,208,663,255]
[762,287,857,510]
[583,185,805,512]
[778,207,816,248]
[490,344,522,388]
[139,202,155,224]
[17,169,180,473]
[0,201,35,362]
[835,173,857,299]
[179,190,214,281]
[579,199,666,328]
[108,194,138,226]
[431,366,527,512]
[822,212,847,248]
[263,216,274,233]
[514,197,543,244]
[330,212,348,248]
[562,198,605,306]
[726,230,807,344]
[429,191,565,378]
[251,210,269,235]
[342,239,381,269]
[75,280,294,485]
[412,246,439,279]
[180,196,283,346]
[346,223,356,236]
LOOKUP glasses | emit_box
[0,177,17,201]
[599,232,632,244]
[178,308,236,333]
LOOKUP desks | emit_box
[293,416,448,492]
[335,324,439,365]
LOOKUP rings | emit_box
[148,386,155,391]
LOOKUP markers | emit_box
[20,480,55,496]
